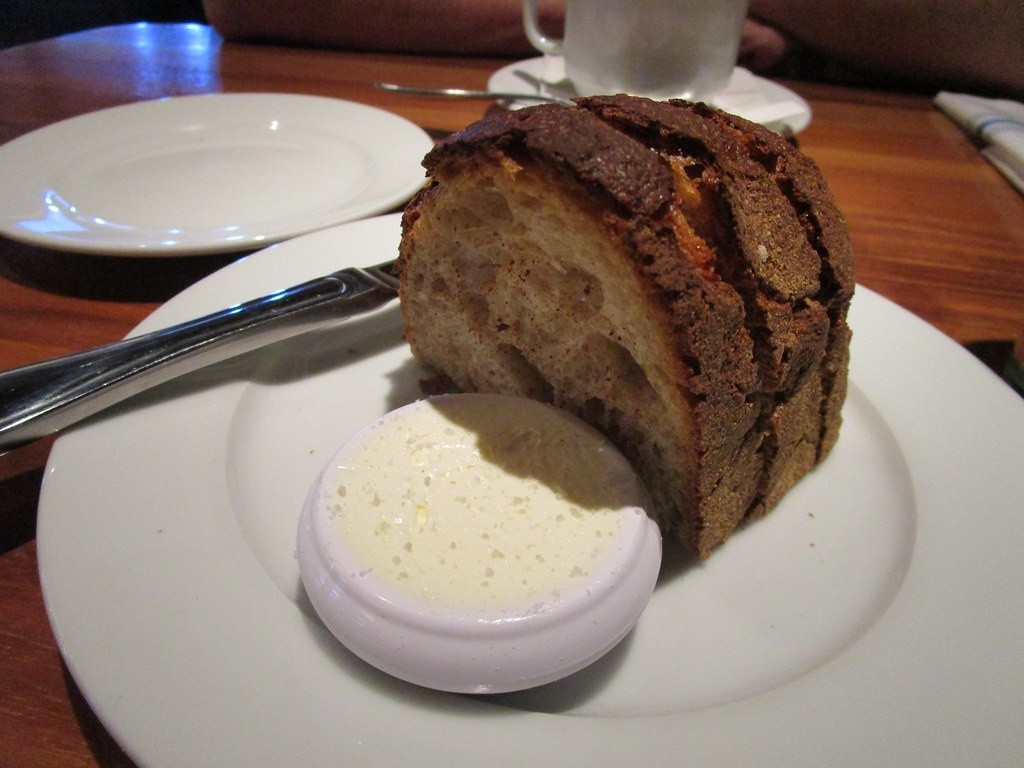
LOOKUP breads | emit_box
[396,96,856,561]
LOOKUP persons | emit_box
[204,1,1024,99]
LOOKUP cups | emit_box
[519,0,748,105]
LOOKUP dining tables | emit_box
[0,20,1024,768]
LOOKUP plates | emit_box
[32,206,1024,768]
[0,95,440,261]
[489,52,812,142]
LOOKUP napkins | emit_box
[933,91,1024,197]
